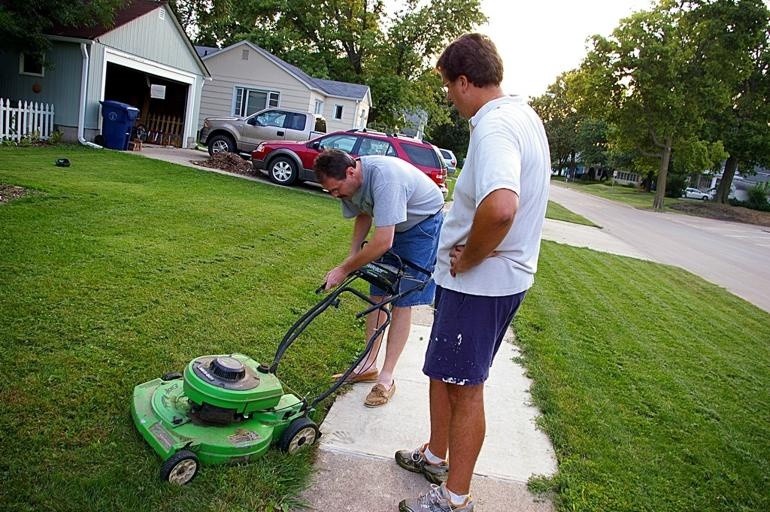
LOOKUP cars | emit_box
[681,187,714,201]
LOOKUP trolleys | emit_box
[125,235,440,493]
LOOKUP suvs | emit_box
[251,125,458,208]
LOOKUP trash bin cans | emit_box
[95,101,140,151]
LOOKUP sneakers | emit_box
[399,481,474,511]
[365,378,396,408]
[395,446,449,483]
[330,369,380,385]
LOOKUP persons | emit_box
[313,146,442,408]
[394,34,555,512]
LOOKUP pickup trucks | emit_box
[196,102,355,160]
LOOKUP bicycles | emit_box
[132,116,149,143]
